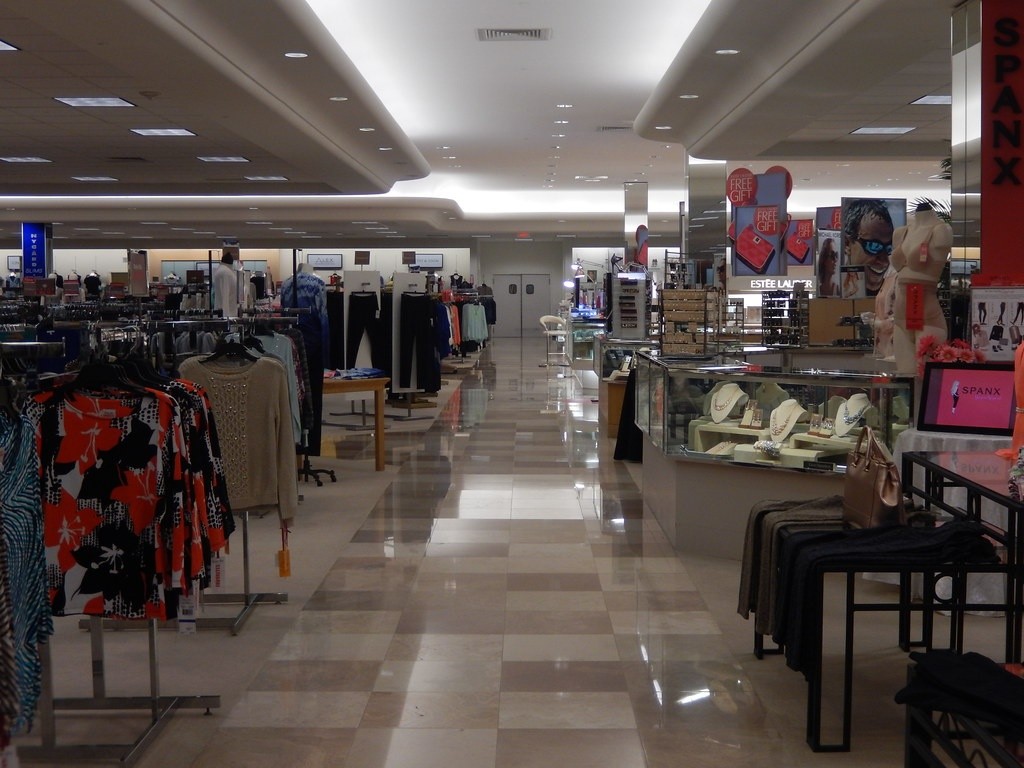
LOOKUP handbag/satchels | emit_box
[842,425,907,530]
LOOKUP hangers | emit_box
[0,282,291,423]
[403,283,428,296]
[449,270,460,276]
[351,283,377,296]
[328,270,339,279]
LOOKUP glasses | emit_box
[856,237,893,255]
[828,251,839,260]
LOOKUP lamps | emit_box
[611,253,653,281]
[570,258,610,293]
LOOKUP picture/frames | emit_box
[917,361,1016,436]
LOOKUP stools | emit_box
[539,315,570,367]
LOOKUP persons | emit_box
[215,253,239,317]
[818,238,840,295]
[845,199,894,296]
[40,270,101,305]
[892,203,953,374]
[717,258,726,289]
[281,263,329,353]
[6,276,20,287]
[844,272,859,296]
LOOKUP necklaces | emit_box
[772,405,797,435]
[714,388,738,411]
[844,402,868,424]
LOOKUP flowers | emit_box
[915,335,986,379]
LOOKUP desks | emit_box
[297,377,391,472]
[902,662,1024,768]
[860,425,1018,617]
[900,449,1024,664]
[599,377,628,438]
[753,505,1019,754]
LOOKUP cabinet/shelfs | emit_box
[665,250,685,289]
[565,319,607,370]
[760,291,810,347]
[658,289,745,359]
